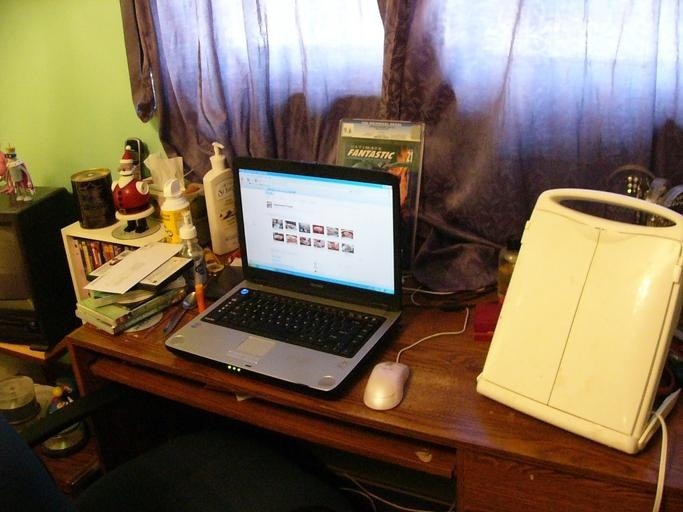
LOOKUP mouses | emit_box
[363,361,409,411]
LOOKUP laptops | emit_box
[165,156,405,401]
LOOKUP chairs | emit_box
[1,384,358,511]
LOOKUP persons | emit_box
[107,145,154,233]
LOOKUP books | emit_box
[72,239,188,335]
[334,117,425,262]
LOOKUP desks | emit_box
[68,270,683,512]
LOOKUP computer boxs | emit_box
[326,452,457,511]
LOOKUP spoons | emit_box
[163,291,198,338]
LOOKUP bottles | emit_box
[495,235,522,303]
[180,223,209,286]
